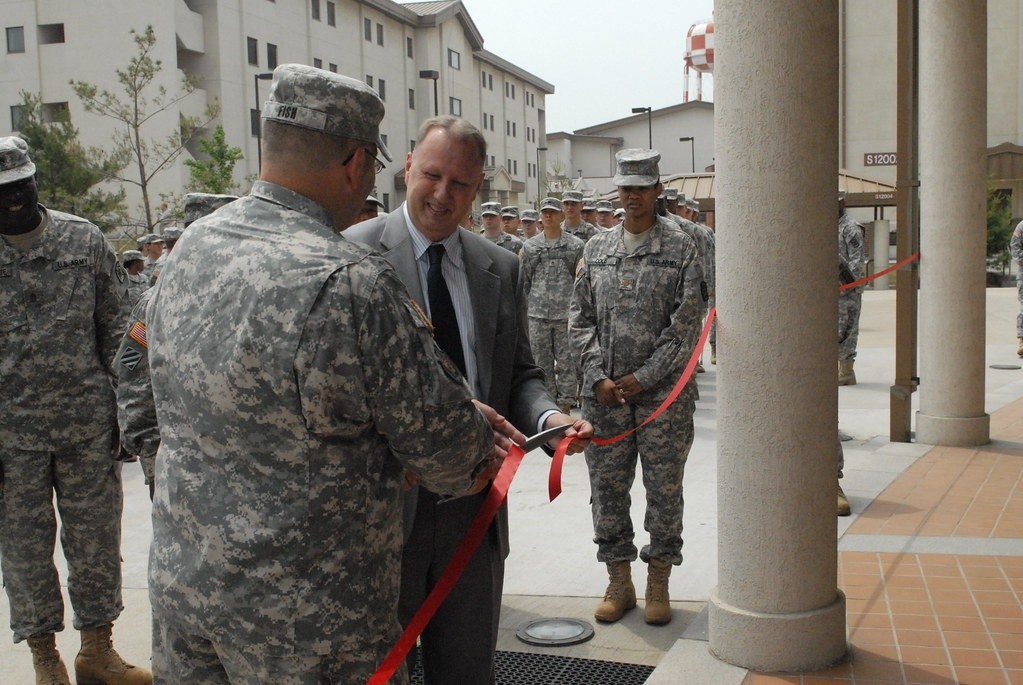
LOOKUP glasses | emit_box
[341,145,387,175]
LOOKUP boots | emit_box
[26,633,69,685]
[644,562,672,625]
[74,623,152,685]
[838,360,856,385]
[594,561,637,624]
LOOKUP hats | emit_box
[481,188,700,217]
[122,192,240,263]
[611,148,661,187]
[262,63,392,163]
[0,135,36,185]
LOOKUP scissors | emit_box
[519,423,573,454]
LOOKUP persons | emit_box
[114,193,238,502]
[837,189,865,386]
[119,61,495,683]
[836,424,851,516]
[337,115,593,685]
[1010,220,1023,358]
[121,203,188,308]
[1,135,155,685]
[568,147,700,625]
[461,187,716,417]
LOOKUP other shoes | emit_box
[837,482,851,516]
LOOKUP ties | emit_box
[425,244,468,383]
